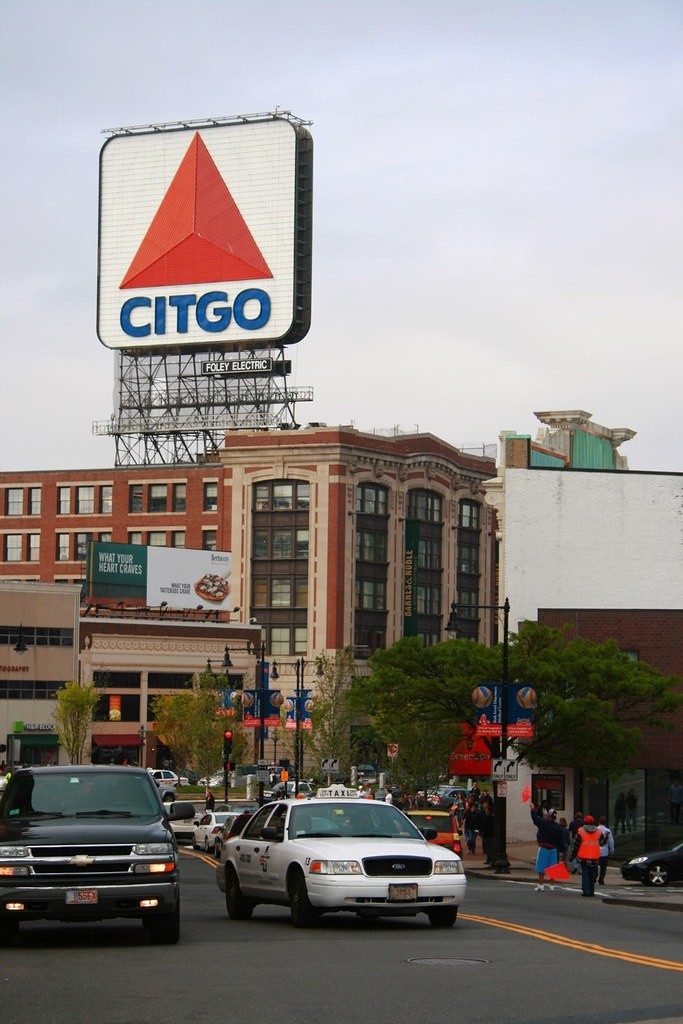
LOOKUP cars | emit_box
[214,783,466,930]
[196,762,320,801]
[146,769,190,788]
[356,784,474,809]
[620,840,683,888]
[151,774,177,803]
[396,809,464,863]
[161,801,258,859]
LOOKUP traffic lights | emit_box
[224,729,234,754]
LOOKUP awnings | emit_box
[122,758,128,764]
[14,735,62,746]
[93,734,146,748]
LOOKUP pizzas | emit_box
[195,573,229,601]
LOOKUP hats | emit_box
[549,808,558,819]
[584,815,595,823]
[359,785,363,789]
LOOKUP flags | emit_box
[521,783,530,803]
[545,861,570,881]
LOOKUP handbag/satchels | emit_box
[451,805,458,812]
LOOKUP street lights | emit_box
[138,724,148,767]
[269,660,300,800]
[298,654,326,780]
[220,642,265,808]
[203,656,229,693]
[442,596,513,874]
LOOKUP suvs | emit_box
[0,765,196,945]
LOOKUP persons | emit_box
[397,792,407,803]
[404,792,415,809]
[275,786,286,800]
[453,783,494,865]
[357,783,393,804]
[595,816,614,885]
[568,811,586,875]
[269,770,276,789]
[668,776,683,824]
[0,761,12,785]
[614,787,640,836]
[530,800,571,891]
[204,790,214,812]
[244,809,249,814]
[570,814,610,896]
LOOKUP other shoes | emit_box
[581,893,594,896]
[549,886,555,892]
[599,881,605,885]
[534,885,545,892]
